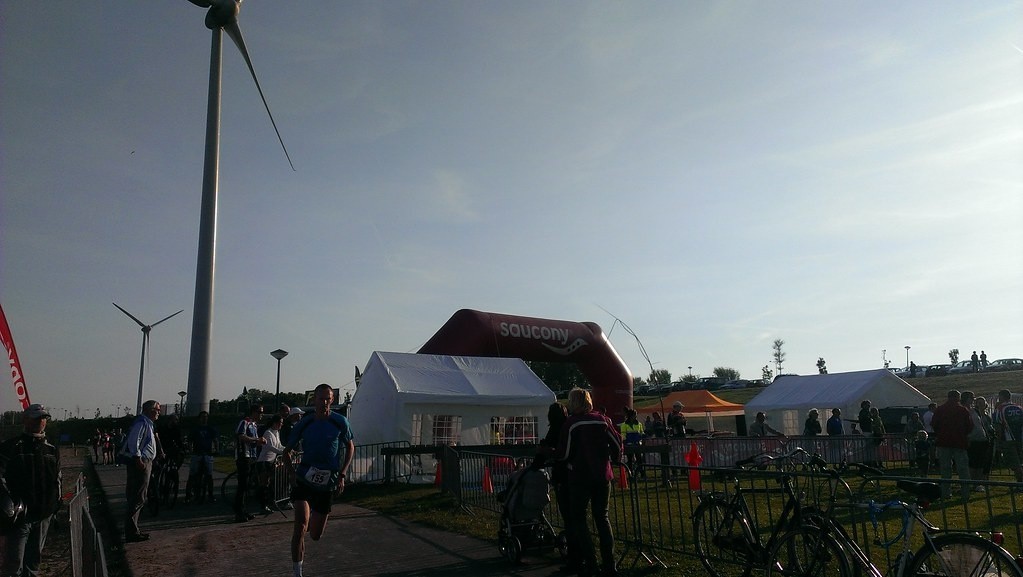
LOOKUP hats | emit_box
[673,401,685,407]
[23,404,51,422]
[290,408,306,417]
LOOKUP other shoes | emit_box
[261,507,275,514]
[236,511,254,522]
[126,532,149,543]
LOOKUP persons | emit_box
[490,415,532,445]
[123,400,305,545]
[282,385,355,577]
[971,351,986,373]
[906,389,1023,500]
[804,400,885,467]
[910,361,917,378]
[0,404,62,577]
[526,389,623,577]
[621,402,688,479]
[93,427,126,467]
[749,410,785,470]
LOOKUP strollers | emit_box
[494,454,571,566]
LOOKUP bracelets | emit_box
[339,473,345,478]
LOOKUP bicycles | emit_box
[688,446,852,577]
[763,461,1023,577]
[220,447,300,515]
[146,440,189,513]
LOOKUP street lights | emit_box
[270,348,288,394]
[178,390,187,410]
[905,346,910,367]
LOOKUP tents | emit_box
[637,390,745,437]
[344,351,558,483]
[743,370,930,465]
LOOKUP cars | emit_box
[947,360,988,376]
[979,359,1023,372]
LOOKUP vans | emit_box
[888,366,928,378]
[924,365,953,378]
